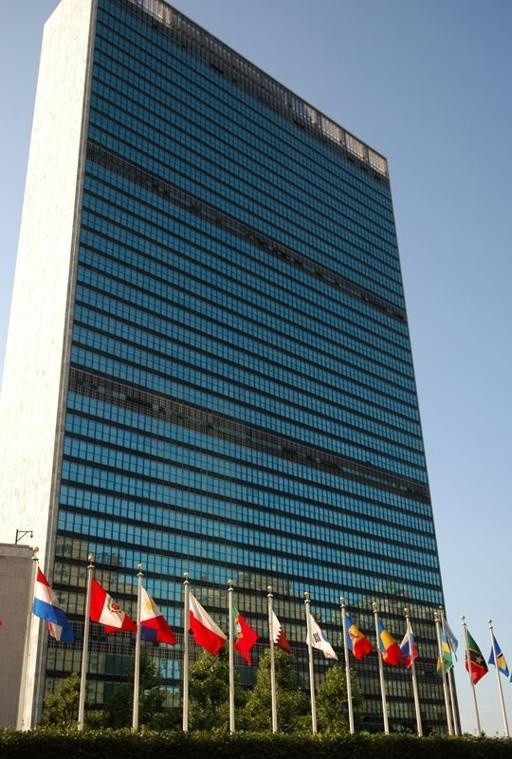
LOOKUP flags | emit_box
[89,577,137,635]
[270,609,294,655]
[436,628,454,678]
[376,618,403,670]
[134,584,178,646]
[305,611,340,661]
[440,615,459,663]
[463,625,489,686]
[30,567,75,644]
[344,614,374,664]
[231,603,260,666]
[397,622,419,670]
[186,591,228,658]
[486,634,512,684]
